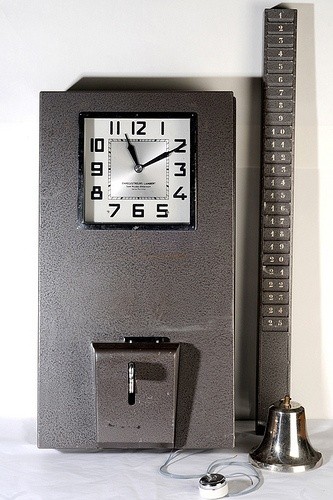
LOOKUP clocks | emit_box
[76,112,197,230]
